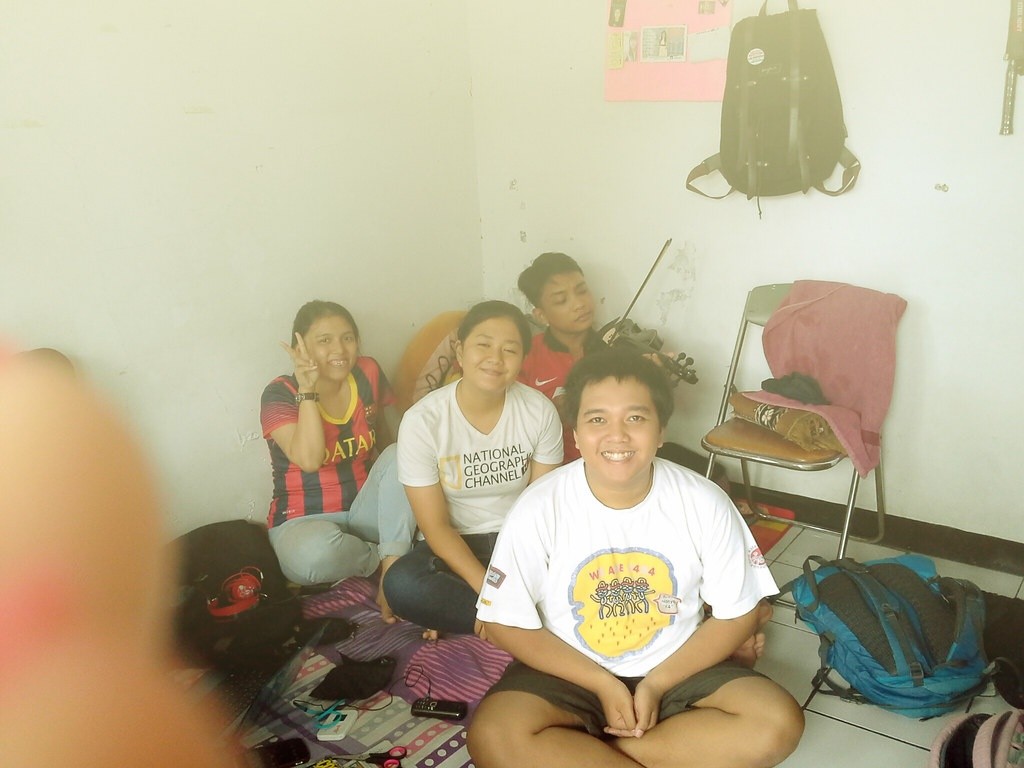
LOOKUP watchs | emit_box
[293,393,320,402]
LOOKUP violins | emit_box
[583,316,698,387]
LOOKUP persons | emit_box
[381,301,563,634]
[657,31,668,55]
[464,348,806,768]
[609,9,623,27]
[260,300,429,628]
[515,251,677,471]
[625,33,638,61]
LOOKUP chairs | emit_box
[701,281,908,561]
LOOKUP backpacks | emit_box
[687,0,863,202]
[156,518,359,668]
[766,547,1023,720]
[926,709,1020,768]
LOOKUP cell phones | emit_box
[257,738,311,768]
[411,698,467,720]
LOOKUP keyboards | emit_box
[196,665,275,735]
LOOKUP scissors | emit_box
[331,746,406,768]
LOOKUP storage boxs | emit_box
[163,520,303,672]
